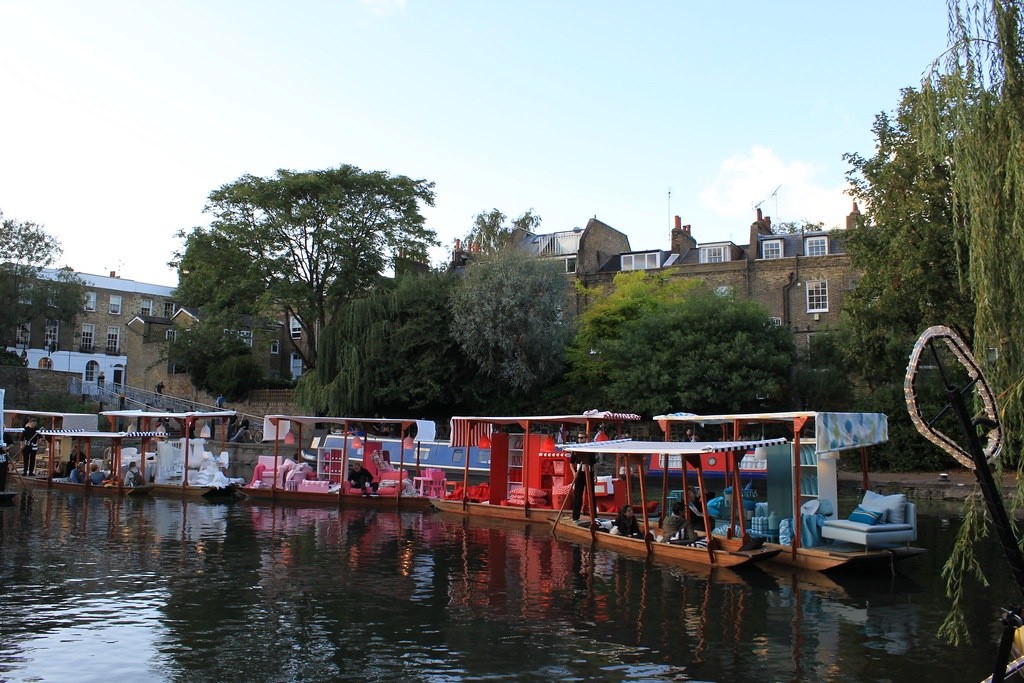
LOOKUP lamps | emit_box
[128,424,136,432]
[200,422,210,437]
[404,427,414,449]
[158,423,166,432]
[285,429,294,444]
[351,427,362,449]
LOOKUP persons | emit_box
[66,444,86,476]
[614,505,644,539]
[97,372,105,389]
[662,501,698,545]
[155,381,164,396]
[90,464,105,485]
[347,463,379,497]
[611,434,617,439]
[20,418,42,476]
[688,486,715,531]
[226,409,251,442]
[597,428,608,441]
[124,462,142,486]
[569,431,597,521]
[70,462,86,483]
[216,395,223,408]
[679,427,691,442]
[0,427,14,492]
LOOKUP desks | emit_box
[413,477,447,496]
[750,533,779,543]
[443,481,464,497]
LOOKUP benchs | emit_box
[822,502,917,555]
[344,471,408,497]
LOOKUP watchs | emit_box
[7,447,10,449]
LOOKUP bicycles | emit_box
[243,422,264,444]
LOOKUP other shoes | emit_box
[370,492,379,496]
[361,492,367,497]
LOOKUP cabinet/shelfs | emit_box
[317,447,344,484]
[539,457,573,490]
[791,438,838,520]
[489,433,555,505]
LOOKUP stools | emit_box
[287,480,298,491]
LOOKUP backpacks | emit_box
[129,468,143,486]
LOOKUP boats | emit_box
[0,408,662,526]
[546,434,787,584]
[652,412,931,575]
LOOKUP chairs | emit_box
[421,468,445,498]
[258,456,282,484]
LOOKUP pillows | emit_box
[849,490,906,525]
[507,487,547,504]
